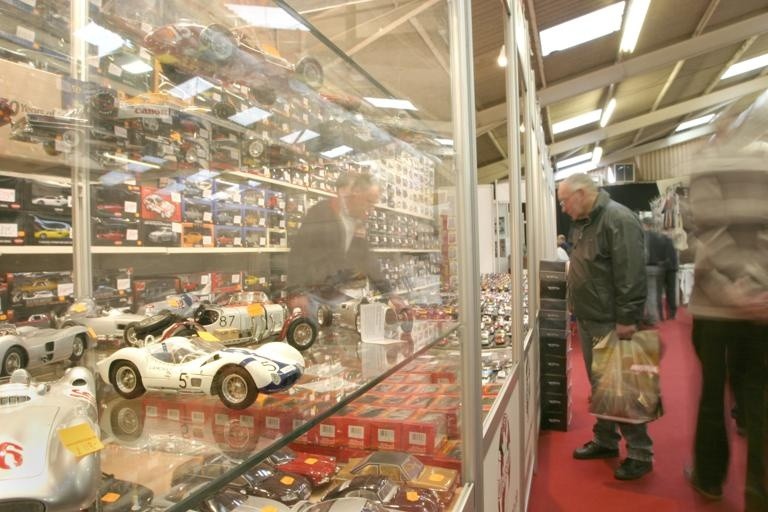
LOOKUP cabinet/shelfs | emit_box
[1,44,443,299]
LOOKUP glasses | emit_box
[560,191,576,207]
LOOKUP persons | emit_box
[685,281,749,499]
[556,233,573,262]
[653,219,681,321]
[556,172,655,481]
[641,213,662,323]
[677,89,767,509]
[286,171,410,312]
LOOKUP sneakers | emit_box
[574,441,619,459]
[615,458,652,480]
[685,466,722,499]
